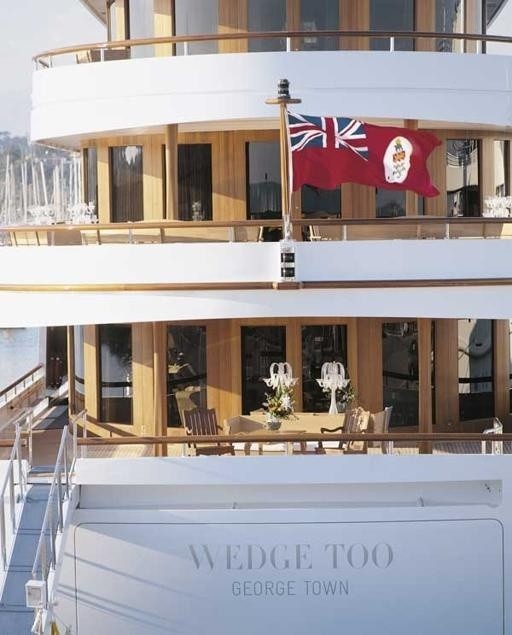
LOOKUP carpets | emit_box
[32,403,69,430]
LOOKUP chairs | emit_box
[314,407,371,455]
[184,407,235,456]
[309,225,333,241]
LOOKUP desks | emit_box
[250,407,344,433]
[240,429,308,456]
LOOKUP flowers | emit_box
[325,386,355,406]
[263,386,297,417]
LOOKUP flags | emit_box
[284,107,442,198]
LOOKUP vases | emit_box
[335,401,348,413]
